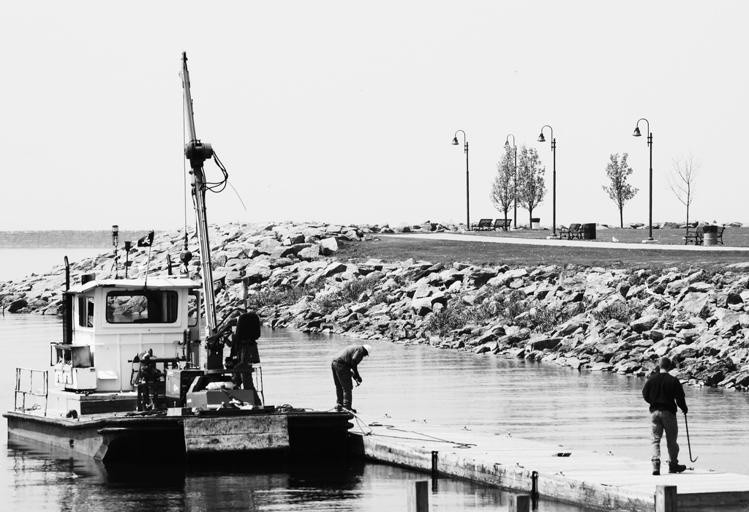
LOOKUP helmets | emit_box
[362,343,373,357]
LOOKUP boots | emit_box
[651,457,660,476]
[669,460,687,474]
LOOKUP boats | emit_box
[3,48,369,487]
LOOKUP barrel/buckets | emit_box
[584,223,596,240]
[703,226,718,246]
[186,391,208,412]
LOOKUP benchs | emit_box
[559,224,584,240]
[494,219,512,231]
[684,226,725,245]
[471,219,493,231]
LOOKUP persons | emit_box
[642,357,688,475]
[331,344,371,413]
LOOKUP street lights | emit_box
[632,117,655,242]
[503,134,519,229]
[537,125,557,237]
[452,130,471,231]
